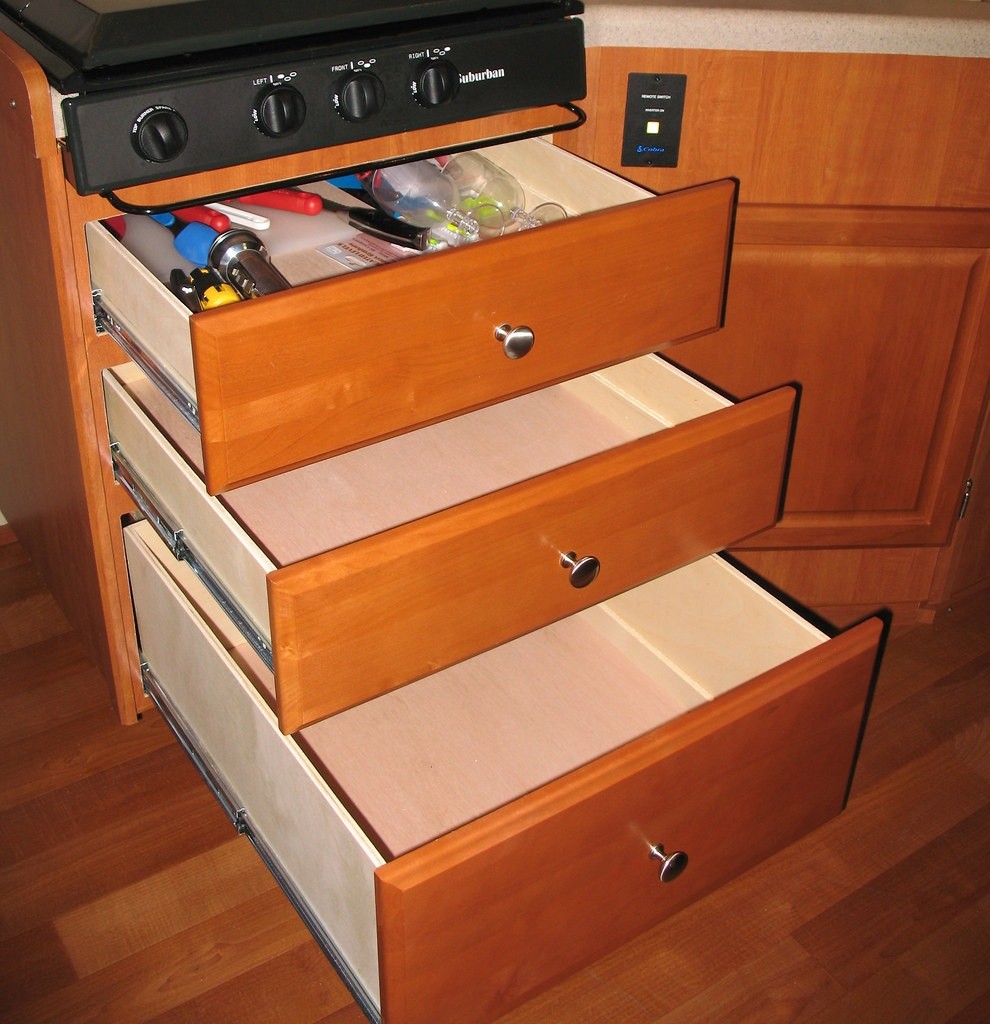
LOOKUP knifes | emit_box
[286,186,430,252]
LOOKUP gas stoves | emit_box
[0,0,592,197]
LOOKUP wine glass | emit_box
[353,166,505,246]
[440,150,568,231]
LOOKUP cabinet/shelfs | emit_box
[0,40,990,1024]
